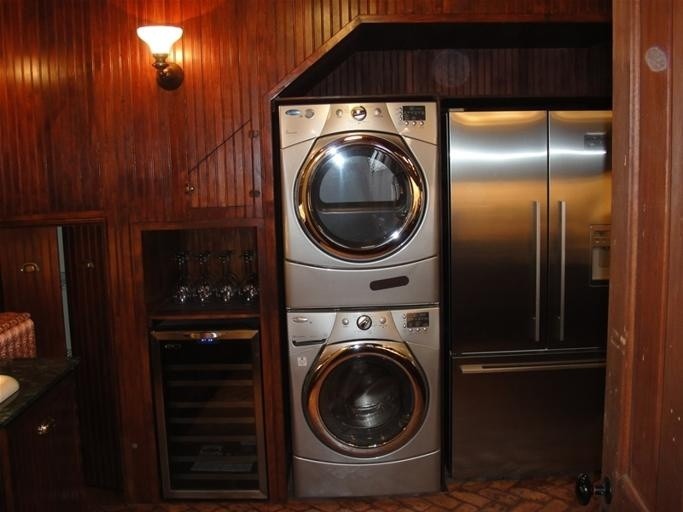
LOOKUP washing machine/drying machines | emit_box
[285,309,444,500]
[274,99,443,309]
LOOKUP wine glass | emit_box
[192,251,217,307]
[215,250,238,306]
[237,251,260,308]
[171,252,192,308]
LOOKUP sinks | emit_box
[0,374,24,413]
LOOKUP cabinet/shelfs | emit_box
[0,374,85,510]
[126,218,279,507]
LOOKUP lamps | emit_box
[133,24,186,90]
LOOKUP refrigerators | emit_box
[441,106,614,484]
